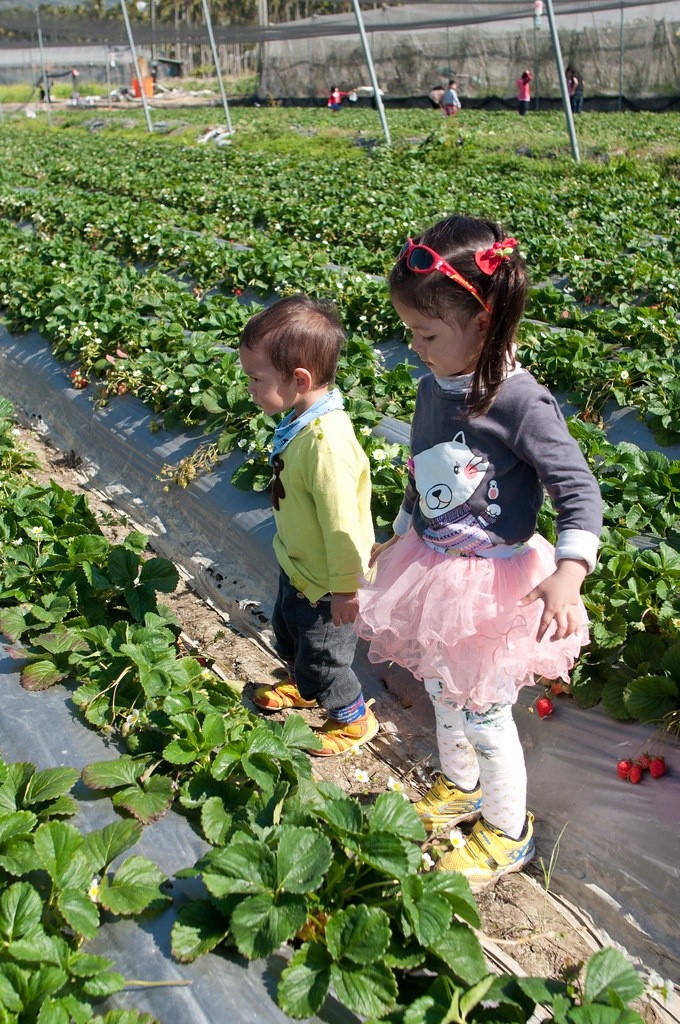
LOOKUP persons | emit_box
[427,87,445,109]
[328,86,356,111]
[516,70,533,116]
[346,217,610,894]
[565,65,585,114]
[348,86,361,108]
[439,80,463,116]
[239,296,381,761]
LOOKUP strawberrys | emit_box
[639,751,654,770]
[649,754,666,778]
[536,696,554,719]
[616,760,631,780]
[630,764,642,783]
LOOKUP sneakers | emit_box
[410,774,536,894]
[253,676,379,758]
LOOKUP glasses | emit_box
[397,238,492,316]
